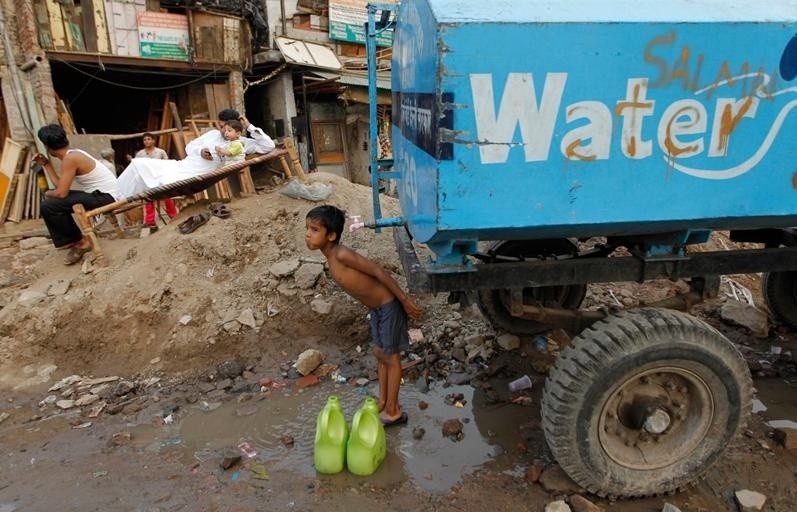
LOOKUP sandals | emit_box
[178,213,211,234]
[209,205,231,217]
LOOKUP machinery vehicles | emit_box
[350,0,797,499]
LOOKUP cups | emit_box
[507,374,533,393]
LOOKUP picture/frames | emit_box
[311,118,350,167]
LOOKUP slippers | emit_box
[379,412,408,428]
[64,246,91,265]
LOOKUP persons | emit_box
[93,148,128,239]
[380,114,396,198]
[217,120,246,167]
[185,109,276,161]
[135,133,180,236]
[31,124,117,265]
[304,204,425,426]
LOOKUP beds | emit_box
[64,136,313,267]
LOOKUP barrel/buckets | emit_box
[313,396,347,475]
[346,398,388,477]
